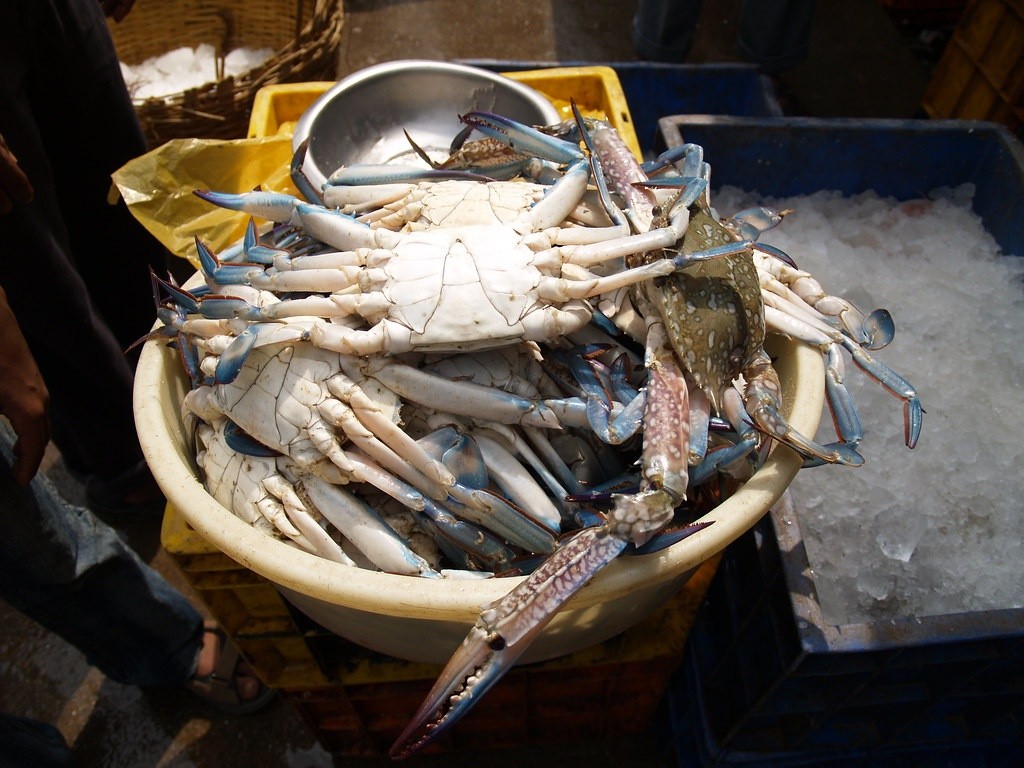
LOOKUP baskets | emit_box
[105,0,345,155]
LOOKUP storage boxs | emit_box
[159,67,723,768]
[453,55,785,160]
[656,109,1024,768]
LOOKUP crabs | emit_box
[121,95,928,761]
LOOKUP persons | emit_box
[0,279,274,768]
[631,0,810,118]
[0,0,192,526]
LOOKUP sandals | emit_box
[182,617,275,715]
